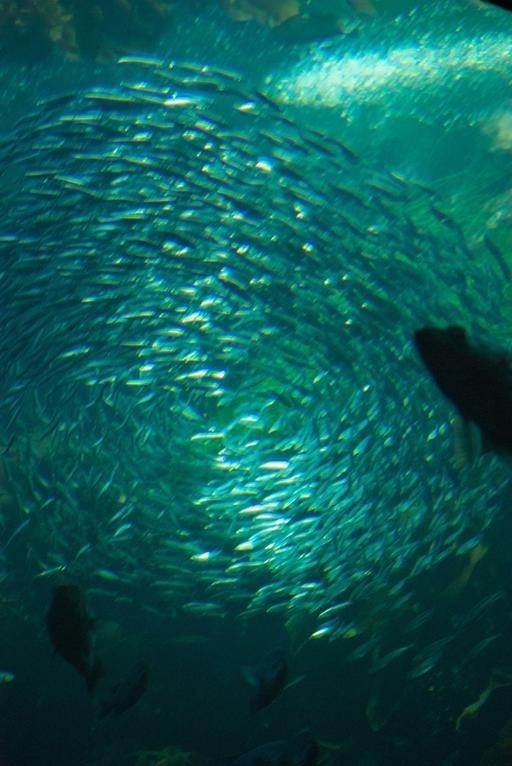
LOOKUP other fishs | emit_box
[0,0,512,766]
